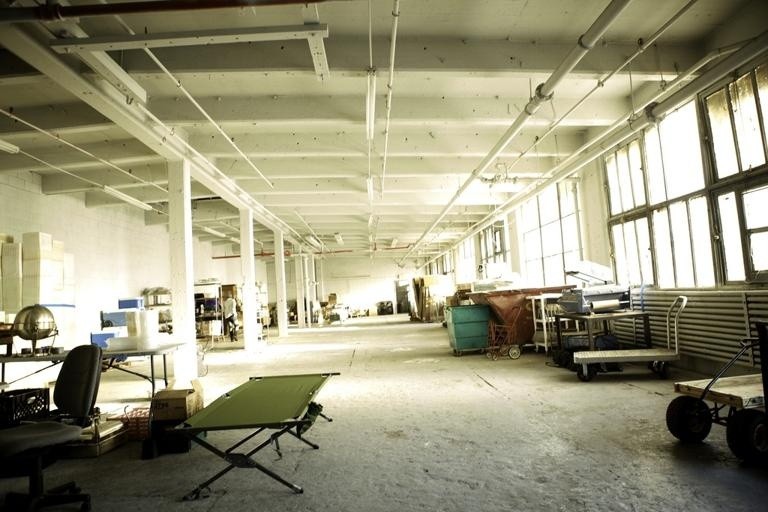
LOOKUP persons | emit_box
[223,289,240,342]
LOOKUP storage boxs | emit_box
[443,305,492,351]
[150,418,209,454]
[152,387,197,420]
[168,376,205,413]
[89,294,144,348]
[0,229,77,313]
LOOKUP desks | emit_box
[551,310,656,365]
[0,340,188,411]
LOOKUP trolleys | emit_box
[485,305,523,361]
[525,293,579,357]
[664,321,767,468]
[572,294,688,380]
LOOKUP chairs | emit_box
[167,367,342,506]
[1,341,104,510]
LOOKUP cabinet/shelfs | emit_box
[524,292,562,354]
[140,281,272,348]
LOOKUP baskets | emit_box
[1,387,49,421]
[106,404,151,440]
